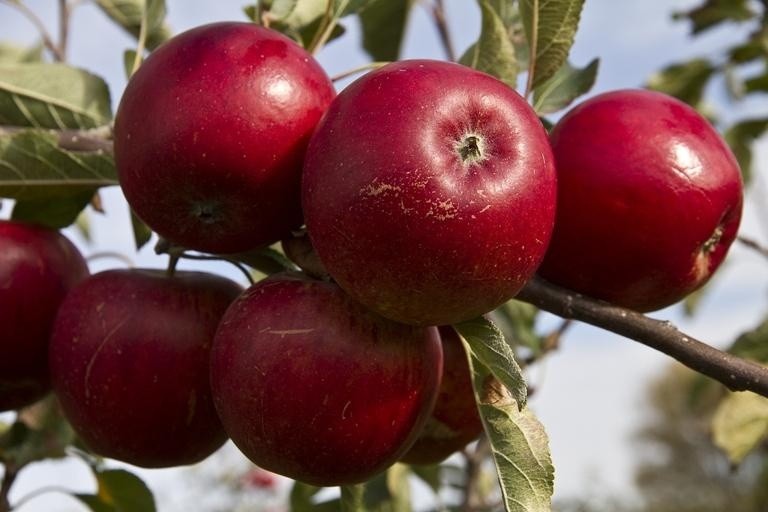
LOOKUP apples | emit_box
[0,220,90,414]
[400,325,484,466]
[45,268,245,469]
[209,270,444,487]
[536,89,744,313]
[113,21,338,255]
[300,60,558,328]
